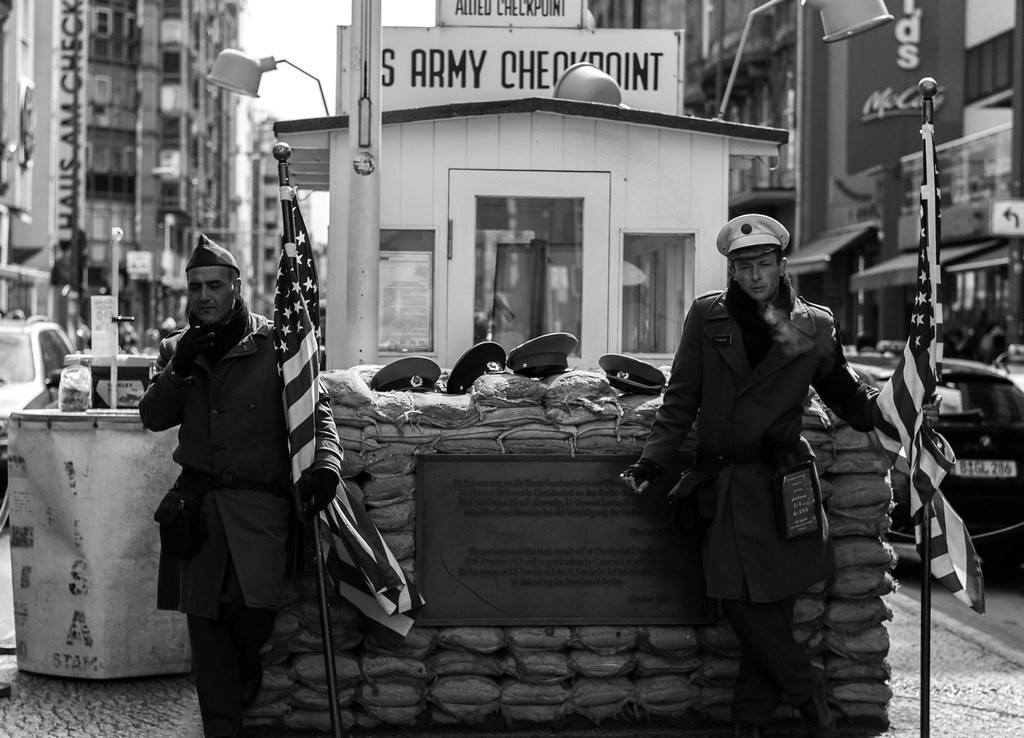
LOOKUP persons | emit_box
[119,314,187,355]
[943,316,1010,374]
[140,234,344,738]
[856,331,881,356]
[0,309,25,320]
[621,213,943,738]
[75,316,90,351]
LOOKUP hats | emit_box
[370,357,441,391]
[717,214,790,263]
[507,332,578,376]
[186,234,240,277]
[599,355,666,396]
[447,342,509,394]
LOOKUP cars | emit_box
[843,352,1024,570]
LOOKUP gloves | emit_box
[298,468,339,521]
[173,320,216,378]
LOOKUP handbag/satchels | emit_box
[155,493,206,558]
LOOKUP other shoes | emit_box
[239,646,261,708]
[798,679,836,738]
[732,721,761,738]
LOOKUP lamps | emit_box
[204,46,331,117]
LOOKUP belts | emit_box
[696,448,769,466]
[206,473,273,492]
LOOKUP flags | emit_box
[273,188,427,649]
[875,125,986,614]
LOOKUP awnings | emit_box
[853,242,991,290]
[786,229,868,274]
[946,243,1024,271]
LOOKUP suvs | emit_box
[0,314,78,495]
